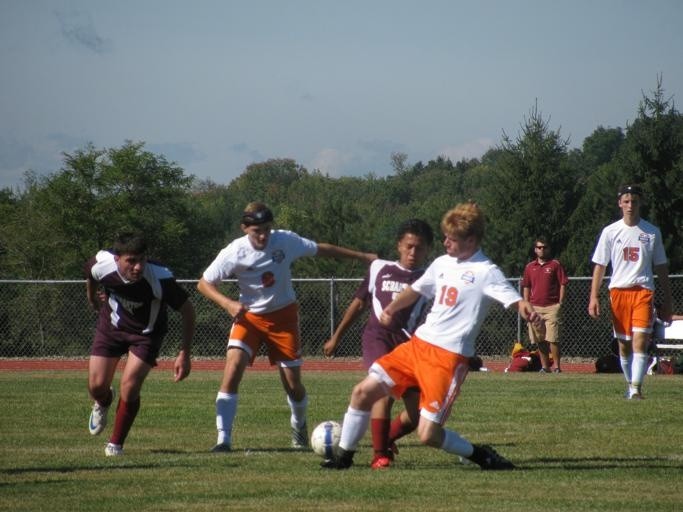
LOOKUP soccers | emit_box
[311,421,342,457]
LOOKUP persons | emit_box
[522,235,570,376]
[82,228,193,456]
[583,183,673,399]
[323,217,433,468]
[315,200,537,471]
[195,202,380,453]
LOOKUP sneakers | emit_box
[88,385,115,436]
[320,443,400,469]
[104,443,124,458]
[480,444,515,469]
[625,387,645,401]
[290,416,309,450]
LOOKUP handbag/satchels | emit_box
[505,351,542,372]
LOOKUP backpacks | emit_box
[594,355,623,374]
